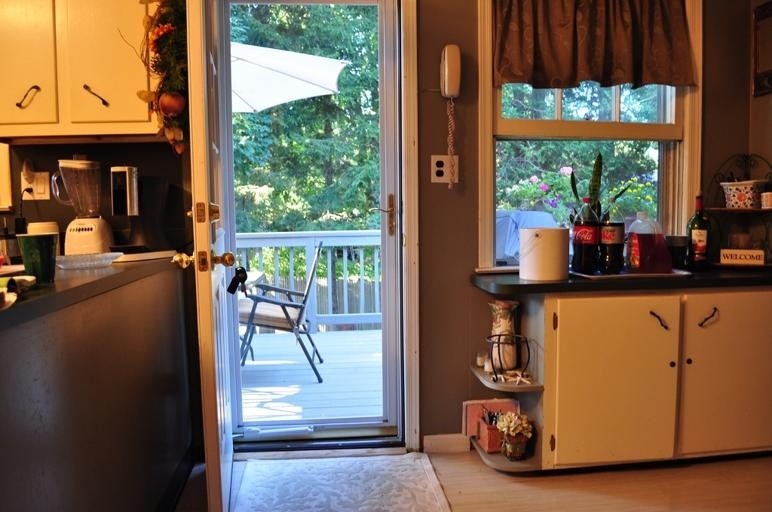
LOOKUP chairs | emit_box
[233,238,327,383]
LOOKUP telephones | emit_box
[440,44,461,97]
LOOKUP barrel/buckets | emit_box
[515,228,569,282]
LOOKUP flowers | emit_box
[494,409,533,439]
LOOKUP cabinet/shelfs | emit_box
[516,290,772,470]
[466,367,541,473]
[1,0,154,125]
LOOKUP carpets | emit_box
[229,450,449,509]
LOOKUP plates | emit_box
[1,292,18,311]
[1,275,38,293]
[0,264,26,275]
[55,250,124,270]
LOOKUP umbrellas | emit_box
[230,41,352,115]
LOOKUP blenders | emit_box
[52,155,117,256]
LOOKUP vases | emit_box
[501,431,529,462]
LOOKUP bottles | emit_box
[570,197,599,274]
[684,194,715,271]
[626,211,672,274]
[598,197,625,274]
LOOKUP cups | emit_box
[16,232,60,286]
